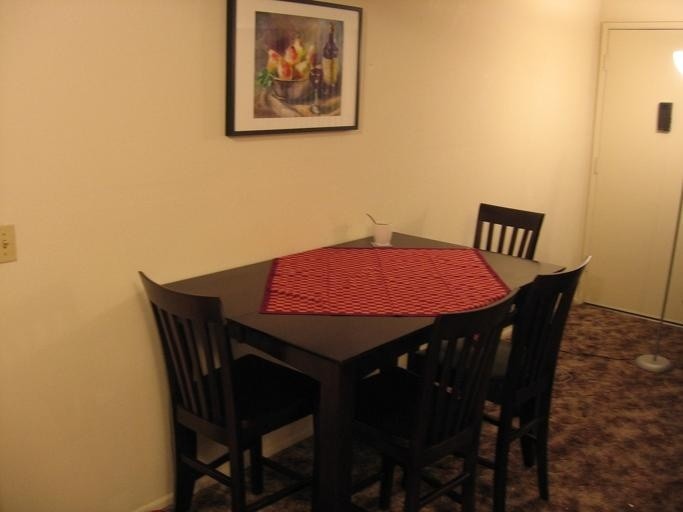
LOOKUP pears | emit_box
[268,33,317,80]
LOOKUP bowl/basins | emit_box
[270,74,312,101]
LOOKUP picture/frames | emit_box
[226,0,362,137]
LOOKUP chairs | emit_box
[474,204,545,260]
[139,272,322,512]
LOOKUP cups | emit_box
[372,223,394,248]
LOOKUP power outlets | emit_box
[0,225,17,264]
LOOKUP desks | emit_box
[162,233,565,512]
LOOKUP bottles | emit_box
[322,21,339,96]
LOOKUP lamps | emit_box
[636,50,683,373]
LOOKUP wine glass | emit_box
[309,68,322,114]
